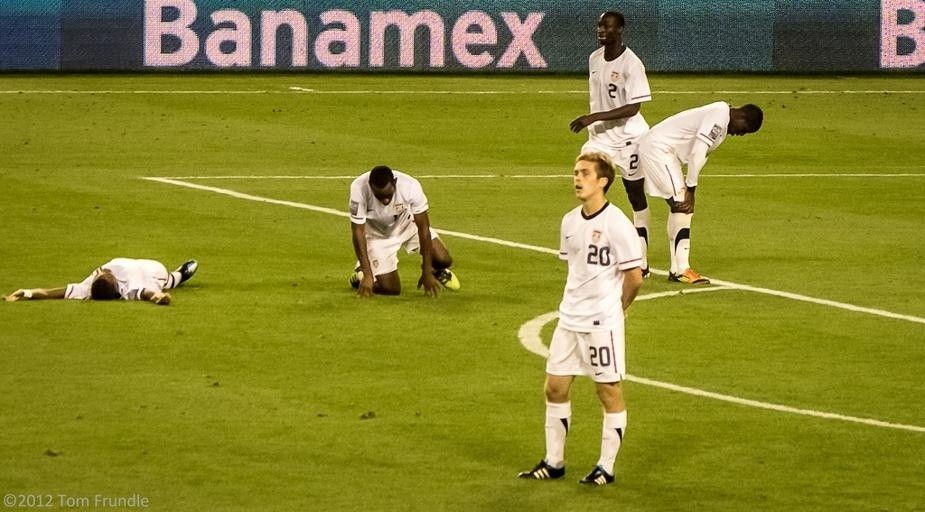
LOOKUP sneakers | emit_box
[578,466,615,487]
[670,267,710,285]
[177,259,198,283]
[350,260,363,290]
[516,460,566,480]
[436,269,463,290]
[642,265,650,278]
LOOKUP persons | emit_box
[518,154,642,486]
[639,101,764,286]
[350,164,461,297]
[0,256,199,304]
[569,12,652,278]
